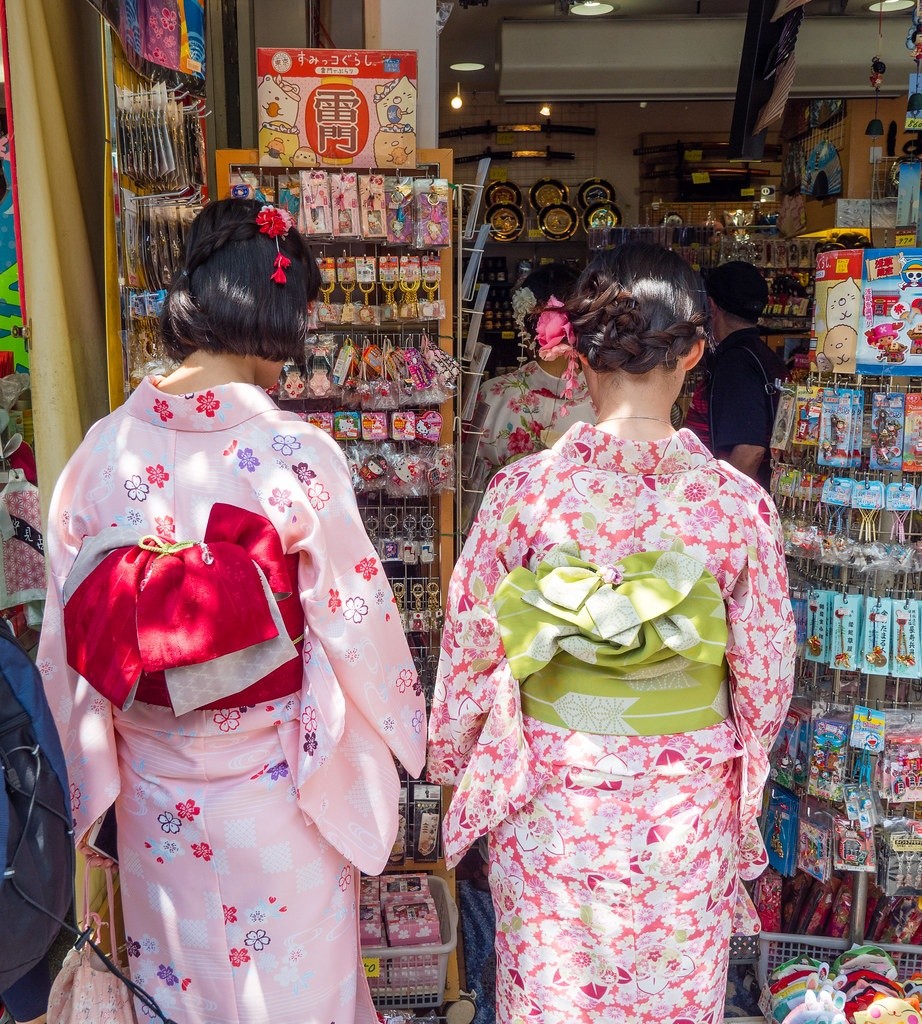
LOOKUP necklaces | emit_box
[598,417,671,422]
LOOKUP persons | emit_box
[425,241,797,1024]
[704,261,786,495]
[461,262,599,551]
[35,199,428,1024]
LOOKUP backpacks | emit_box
[1,617,176,1024]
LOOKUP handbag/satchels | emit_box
[46,854,138,1024]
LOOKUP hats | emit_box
[705,261,768,316]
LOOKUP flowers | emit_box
[532,293,582,419]
[255,203,296,283]
[511,286,536,370]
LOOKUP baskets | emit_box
[360,874,459,1010]
[728,933,759,966]
[754,931,922,994]
[641,199,780,225]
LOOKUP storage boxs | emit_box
[456,255,514,332]
